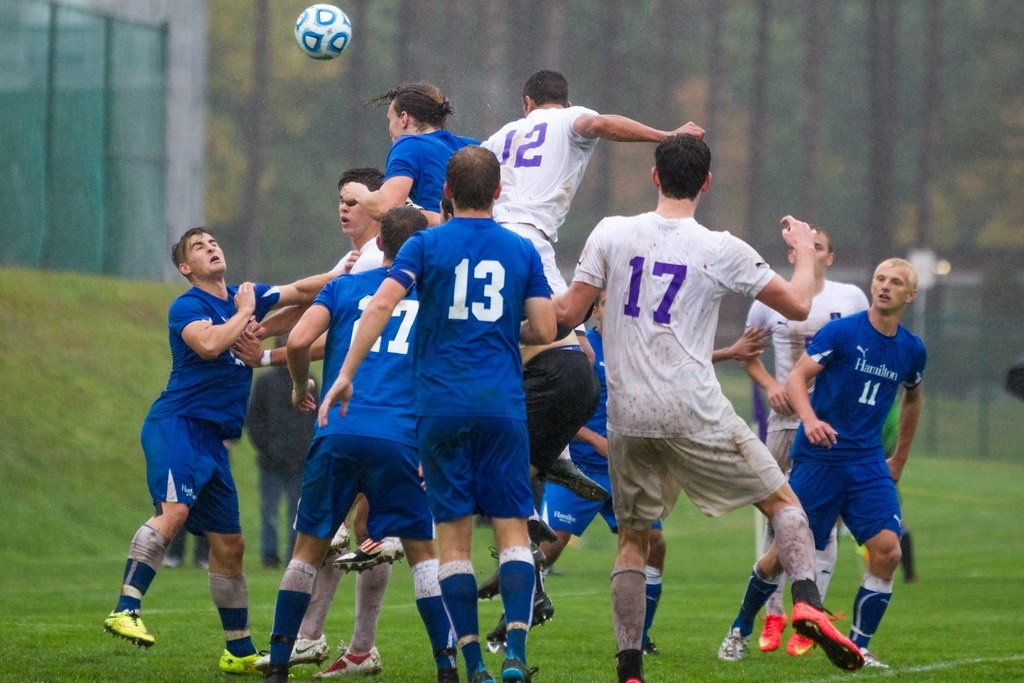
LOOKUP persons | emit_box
[232,70,774,676]
[247,334,319,566]
[162,529,211,569]
[718,257,925,668]
[264,206,460,683]
[104,229,359,673]
[317,145,556,683]
[519,133,865,683]
[744,224,870,656]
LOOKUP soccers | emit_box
[294,4,351,60]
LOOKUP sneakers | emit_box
[716,626,752,663]
[328,522,352,557]
[486,637,504,655]
[529,503,558,543]
[487,589,555,643]
[757,611,787,652]
[859,646,889,669]
[502,657,537,683]
[253,633,330,677]
[641,639,659,657]
[791,604,865,670]
[217,649,265,675]
[333,532,407,574]
[311,638,381,680]
[472,669,495,683]
[543,456,608,501]
[477,541,546,600]
[102,608,155,650]
[787,626,815,657]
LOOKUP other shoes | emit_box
[266,665,289,683]
[437,671,458,683]
[163,554,181,567]
